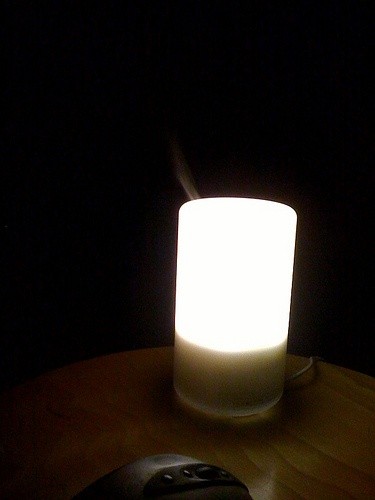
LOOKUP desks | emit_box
[0,343,375,500]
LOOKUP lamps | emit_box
[171,196,298,428]
